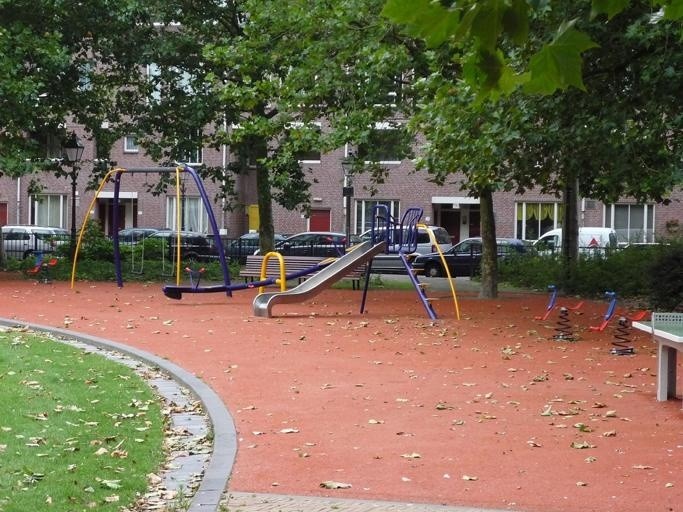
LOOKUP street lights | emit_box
[340,150,360,246]
[62,129,85,261]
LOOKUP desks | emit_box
[632,313,683,402]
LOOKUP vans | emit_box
[532,226,620,261]
[0,225,56,262]
[356,226,453,276]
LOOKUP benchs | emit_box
[239,256,367,291]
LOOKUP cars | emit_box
[45,227,71,251]
[616,241,670,251]
[224,230,293,262]
[110,228,157,246]
[412,238,537,277]
[253,230,362,259]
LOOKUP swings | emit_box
[130,171,148,277]
[159,172,179,279]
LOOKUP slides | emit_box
[253,241,388,318]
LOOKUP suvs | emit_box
[136,229,224,266]
